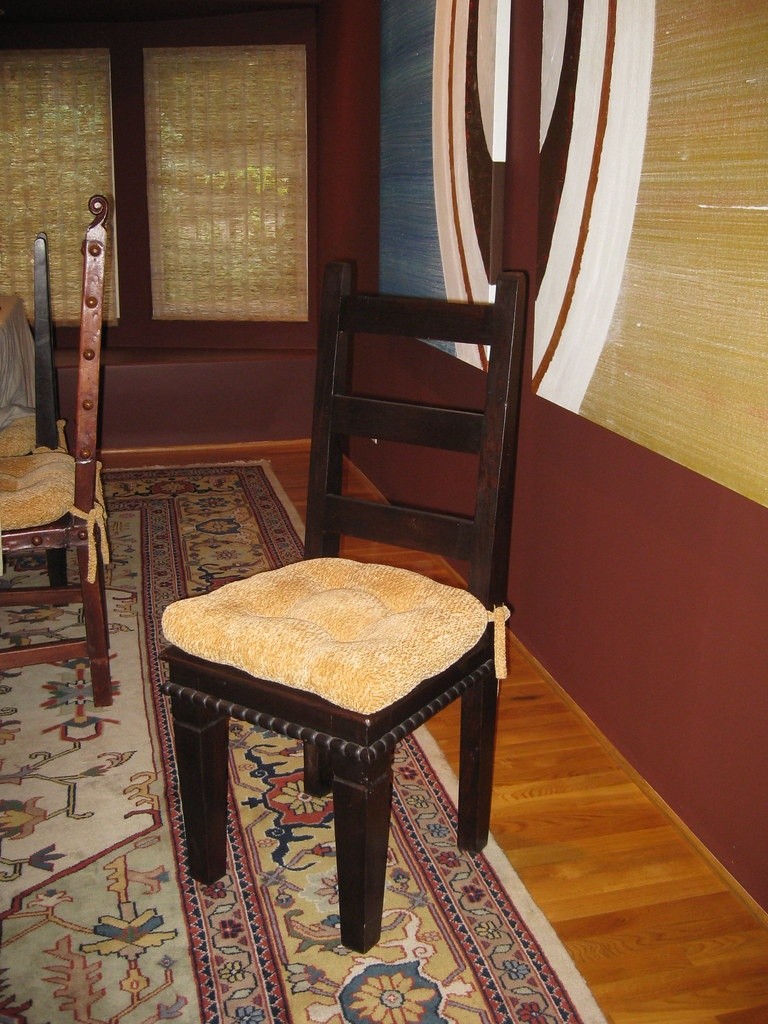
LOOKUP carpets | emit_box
[0,459,601,1024]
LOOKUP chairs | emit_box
[160,261,529,954]
[0,195,113,709]
[0,232,69,608]
